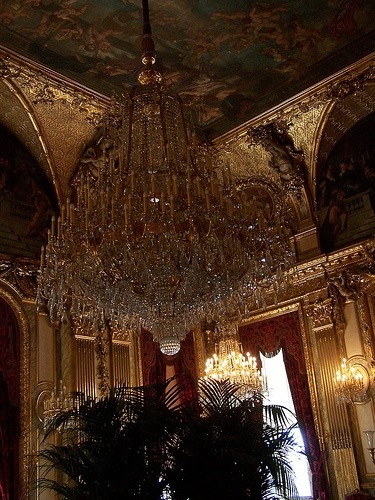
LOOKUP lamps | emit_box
[333,357,364,405]
[37,23,299,355]
[198,341,269,397]
[43,380,83,434]
